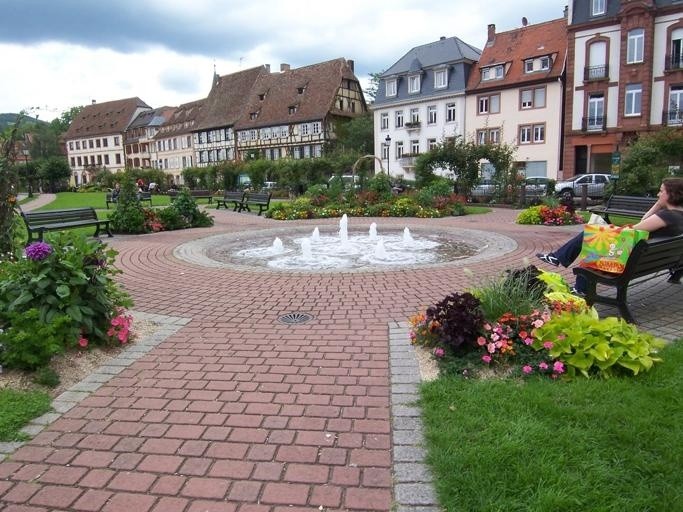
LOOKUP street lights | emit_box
[384,134,392,176]
[21,144,33,197]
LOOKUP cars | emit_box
[519,177,556,196]
[238,179,279,193]
[465,177,505,204]
[327,174,368,190]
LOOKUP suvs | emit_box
[553,173,617,199]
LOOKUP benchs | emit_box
[589,194,660,223]
[165,188,214,206]
[19,207,114,245]
[568,231,682,323]
[105,191,152,210]
[237,193,271,216]
[215,191,243,210]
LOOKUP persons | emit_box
[137,178,144,190]
[292,188,298,199]
[170,179,176,188]
[287,185,293,200]
[390,180,405,197]
[535,177,682,299]
[110,183,128,210]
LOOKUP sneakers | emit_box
[569,286,596,297]
[536,251,561,268]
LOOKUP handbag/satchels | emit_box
[578,225,649,274]
